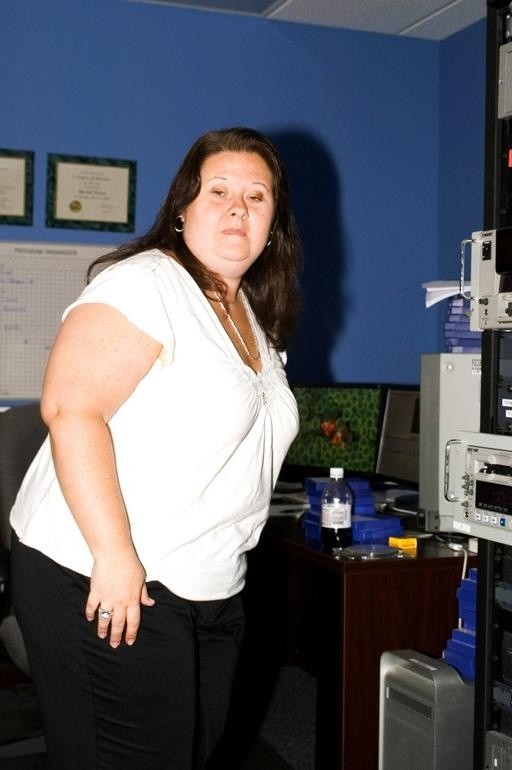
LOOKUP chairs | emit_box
[2,402,50,548]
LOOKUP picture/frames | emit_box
[43,154,138,234]
[0,149,36,227]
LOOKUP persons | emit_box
[9,127,304,769]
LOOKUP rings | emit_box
[98,610,112,619]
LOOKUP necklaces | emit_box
[215,291,260,361]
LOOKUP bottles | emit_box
[321,467,351,560]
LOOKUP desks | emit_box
[268,464,478,770]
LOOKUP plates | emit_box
[341,544,399,560]
